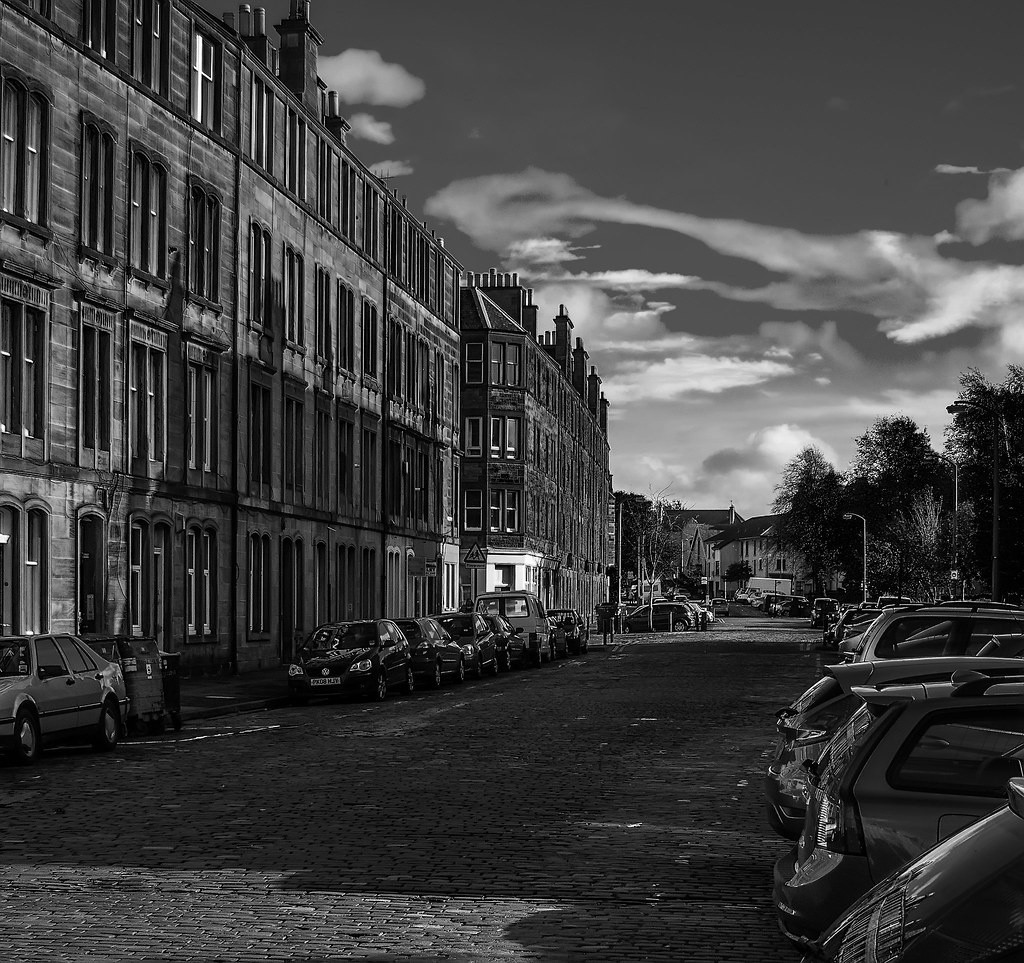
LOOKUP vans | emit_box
[762,593,809,613]
[472,590,556,668]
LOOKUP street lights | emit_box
[947,400,1002,599]
[843,512,868,601]
[922,450,959,601]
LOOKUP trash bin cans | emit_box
[159,649,185,731]
[595,601,618,634]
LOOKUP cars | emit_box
[545,605,587,657]
[480,614,527,671]
[709,597,730,618]
[625,592,715,632]
[736,588,810,616]
[764,596,1023,962]
[0,634,131,763]
[390,618,468,691]
[811,599,841,627]
[430,613,499,677]
[288,617,416,703]
